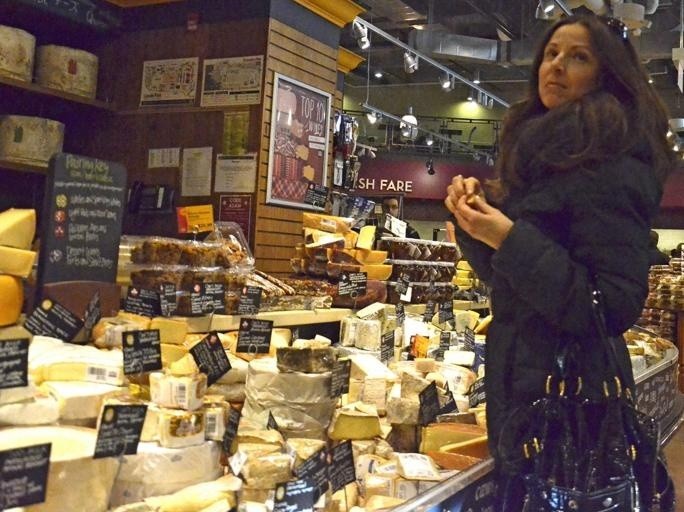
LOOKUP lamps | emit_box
[351,18,495,166]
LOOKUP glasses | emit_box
[596,15,630,47]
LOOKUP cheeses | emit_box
[0,207,670,512]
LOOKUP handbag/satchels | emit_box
[491,391,676,512]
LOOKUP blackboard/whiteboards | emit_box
[39,148,129,284]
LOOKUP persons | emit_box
[442,8,677,509]
[444,212,458,245]
[372,194,423,239]
[648,229,672,266]
